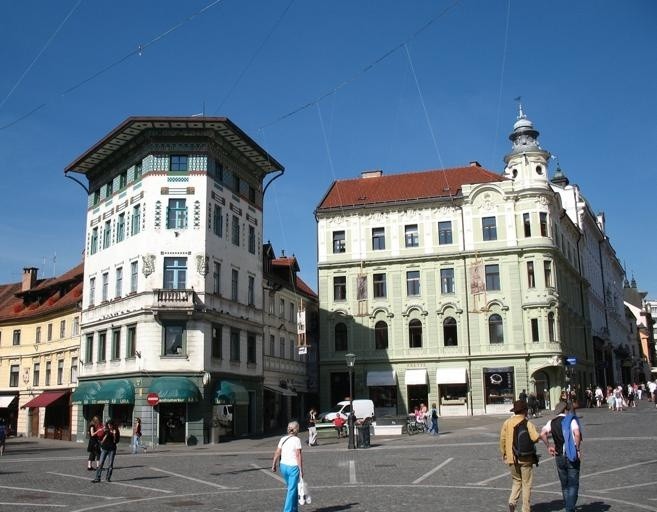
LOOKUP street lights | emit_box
[344,353,360,450]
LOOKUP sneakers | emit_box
[106,477,111,482]
[91,479,101,483]
[144,446,147,454]
[509,503,515,512]
[131,451,137,454]
[88,467,97,471]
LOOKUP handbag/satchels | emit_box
[137,432,142,437]
[298,480,312,505]
[432,410,438,419]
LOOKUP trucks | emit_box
[212,404,233,422]
[317,397,374,423]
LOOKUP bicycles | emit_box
[399,414,426,436]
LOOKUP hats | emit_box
[510,400,529,412]
[555,403,570,415]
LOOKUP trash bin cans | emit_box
[354,416,373,449]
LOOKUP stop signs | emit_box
[146,392,159,406]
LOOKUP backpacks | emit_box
[512,419,538,466]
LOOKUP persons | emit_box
[307,410,321,447]
[332,412,347,439]
[0,419,7,456]
[90,420,121,483]
[420,402,431,429]
[500,400,540,512]
[429,402,440,436]
[412,406,424,427]
[85,417,106,472]
[272,421,304,512]
[132,418,147,454]
[516,380,657,420]
[539,402,583,512]
